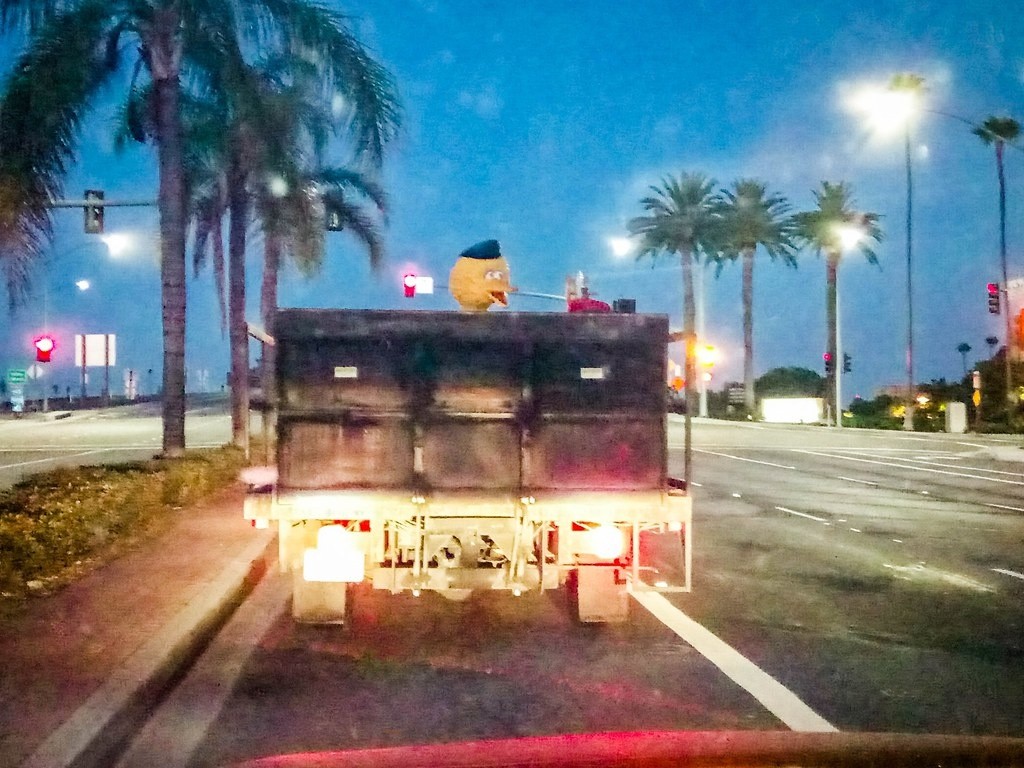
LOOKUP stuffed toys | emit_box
[447,238,519,314]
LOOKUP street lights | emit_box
[832,220,870,426]
[848,79,1019,430]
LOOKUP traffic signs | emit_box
[7,368,26,384]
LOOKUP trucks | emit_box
[240,296,698,629]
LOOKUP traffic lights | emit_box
[35,335,54,362]
[824,351,833,375]
[843,353,852,373]
[405,274,417,297]
[986,282,1000,314]
